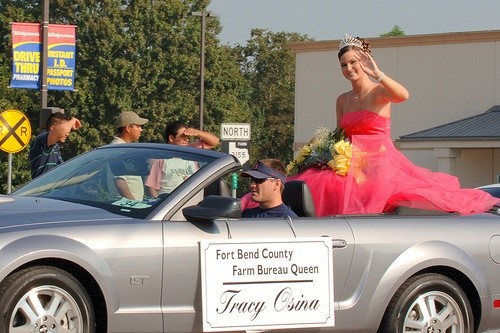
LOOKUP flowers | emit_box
[286,127,368,185]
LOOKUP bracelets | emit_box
[376,72,384,82]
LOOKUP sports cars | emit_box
[0,143,499,333]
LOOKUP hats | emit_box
[115,111,148,128]
[241,160,286,184]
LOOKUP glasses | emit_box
[248,177,275,184]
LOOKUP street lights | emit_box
[190,11,219,131]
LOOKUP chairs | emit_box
[282,180,316,217]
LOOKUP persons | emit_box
[30,112,81,179]
[144,121,219,199]
[241,159,300,218]
[107,111,148,201]
[240,34,500,218]
